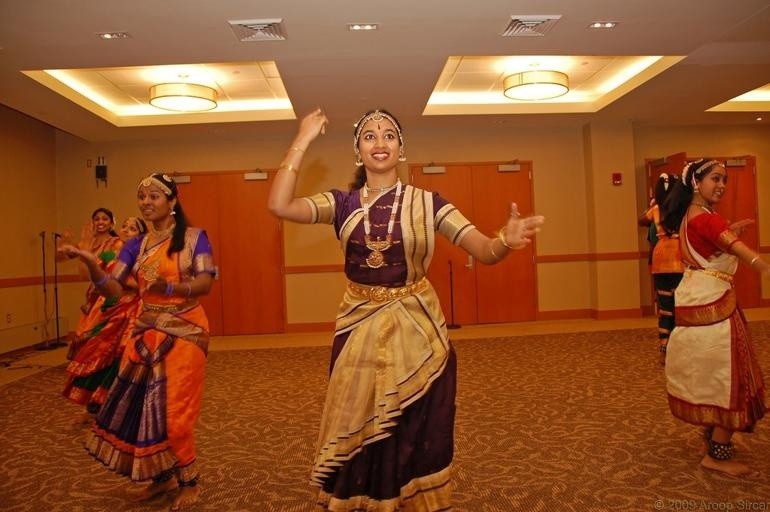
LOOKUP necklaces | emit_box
[689,200,719,214]
[148,222,177,241]
[360,175,402,193]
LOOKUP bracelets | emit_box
[98,274,110,286]
[273,164,300,175]
[279,147,307,155]
[186,281,193,297]
[748,254,762,269]
[498,227,517,252]
[93,273,107,284]
[163,281,170,297]
[488,238,504,263]
[168,283,177,298]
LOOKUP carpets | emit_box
[1,318,770,512]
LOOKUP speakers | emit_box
[96,164,108,179]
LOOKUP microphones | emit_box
[40,231,45,236]
[52,232,60,237]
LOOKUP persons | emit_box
[59,214,150,413]
[269,101,547,511]
[666,156,770,479]
[56,209,127,363]
[637,171,686,366]
[64,173,222,511]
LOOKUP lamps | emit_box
[148,83,218,112]
[503,70,569,101]
